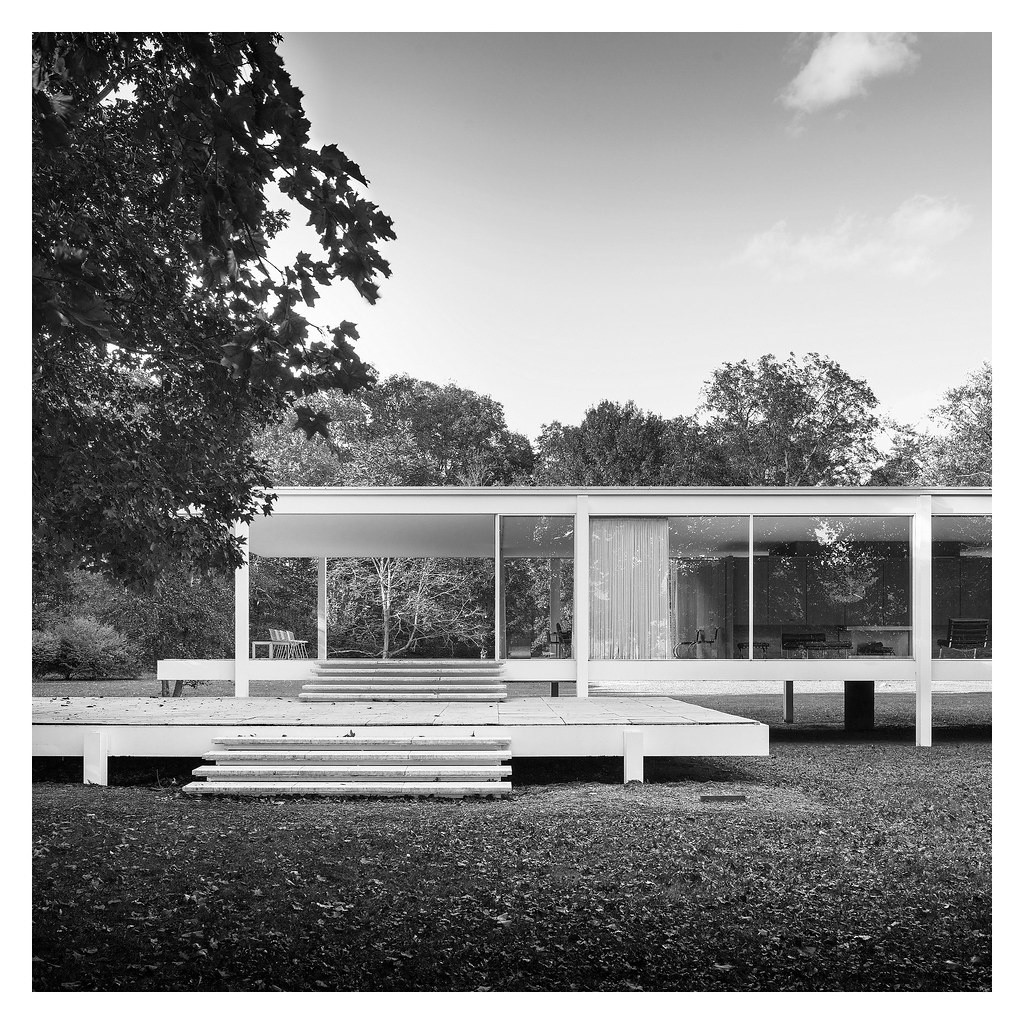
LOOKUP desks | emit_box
[846,625,911,658]
[252,640,291,659]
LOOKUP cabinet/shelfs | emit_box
[933,557,992,625]
[732,557,911,626]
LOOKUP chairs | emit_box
[781,632,826,659]
[547,623,572,659]
[270,629,309,660]
[937,616,989,658]
